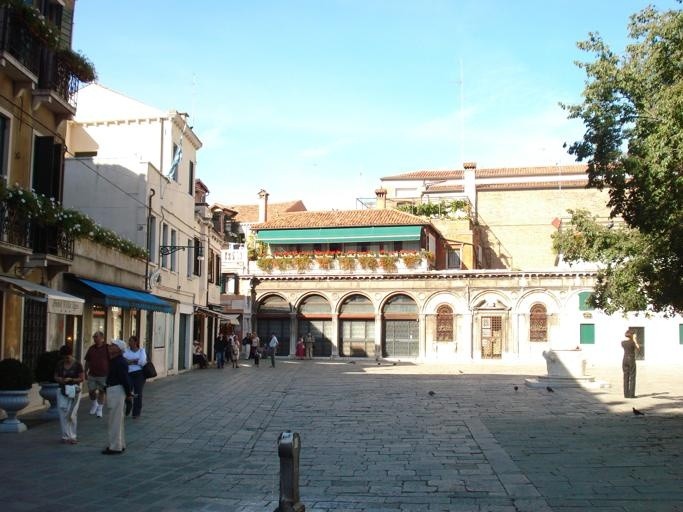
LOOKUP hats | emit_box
[110,338,126,354]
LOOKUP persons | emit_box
[52,344,83,445]
[99,338,133,455]
[191,330,279,370]
[124,336,148,419]
[82,330,110,419]
[295,334,304,360]
[304,332,315,360]
[620,328,640,398]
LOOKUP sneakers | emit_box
[89,399,97,415]
[96,404,103,417]
[105,447,125,451]
[63,438,80,444]
[100,448,123,455]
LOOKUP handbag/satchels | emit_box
[233,349,238,356]
[61,385,80,397]
[142,362,158,379]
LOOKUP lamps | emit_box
[160,244,204,261]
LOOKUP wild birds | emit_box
[514,386,518,391]
[632,407,644,418]
[429,391,436,397]
[547,386,554,393]
